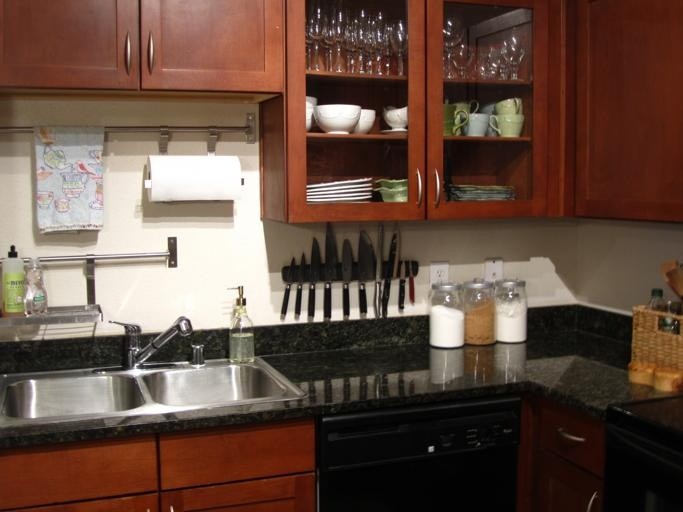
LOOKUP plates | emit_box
[379,129,408,134]
[307,177,373,202]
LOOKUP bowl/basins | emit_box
[356,110,376,134]
[312,104,360,134]
[382,105,408,129]
[306,96,318,129]
[373,178,408,202]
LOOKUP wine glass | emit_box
[305,0,407,75]
[443,3,527,80]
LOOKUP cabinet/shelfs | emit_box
[520,395,602,512]
[560,1,683,224]
[0,0,285,105]
[0,421,316,512]
[260,0,564,224]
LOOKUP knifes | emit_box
[356,230,377,320]
[373,224,386,319]
[381,228,403,319]
[278,256,295,320]
[308,237,322,322]
[341,239,355,321]
[322,222,339,322]
[397,258,407,309]
[295,251,307,320]
[408,258,416,305]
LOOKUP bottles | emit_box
[428,282,464,348]
[462,280,496,345]
[493,278,528,343]
[643,287,668,331]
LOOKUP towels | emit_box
[31,124,108,235]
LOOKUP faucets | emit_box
[108,315,192,369]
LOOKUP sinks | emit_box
[142,359,309,410]
[0,367,145,428]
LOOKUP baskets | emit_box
[629,305,683,393]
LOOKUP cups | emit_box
[453,99,479,134]
[489,114,524,138]
[494,98,523,114]
[443,103,468,136]
[461,113,489,136]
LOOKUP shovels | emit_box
[661,260,683,299]
